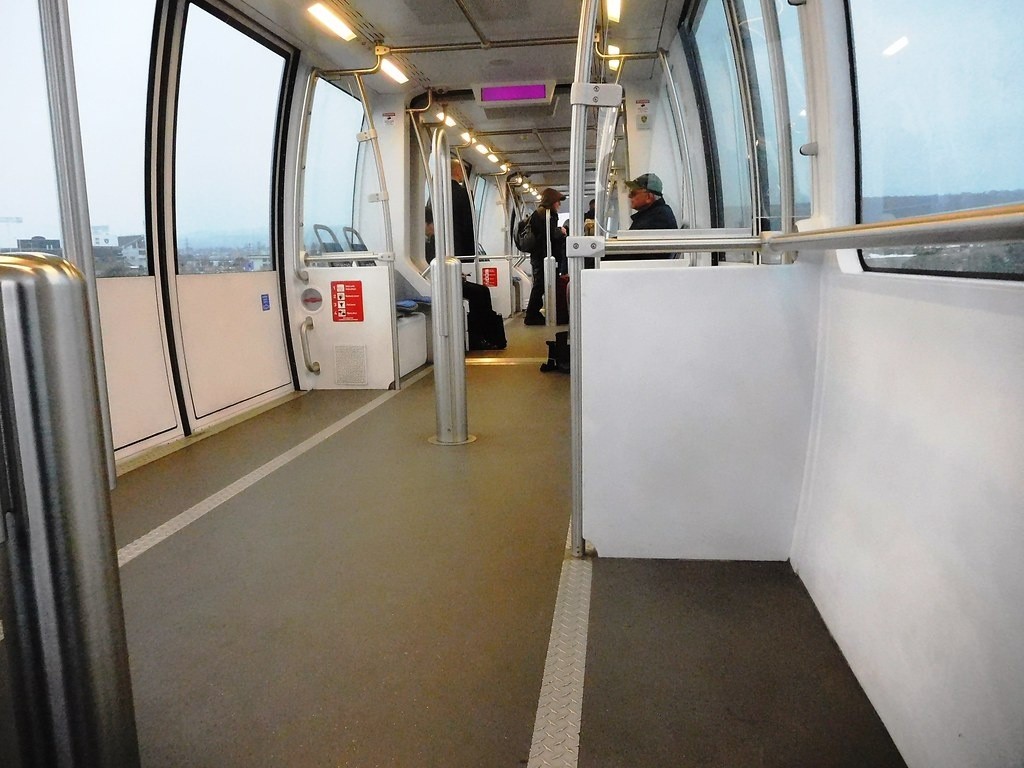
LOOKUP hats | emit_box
[625,173,663,193]
[540,188,566,207]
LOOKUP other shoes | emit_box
[523,312,545,325]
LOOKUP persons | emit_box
[584,199,596,223]
[583,172,679,269]
[451,158,481,262]
[425,207,499,350]
[523,188,568,326]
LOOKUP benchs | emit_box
[314,224,434,380]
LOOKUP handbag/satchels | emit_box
[514,216,543,253]
[466,311,507,350]
[540,332,570,375]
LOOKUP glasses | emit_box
[628,191,648,198]
